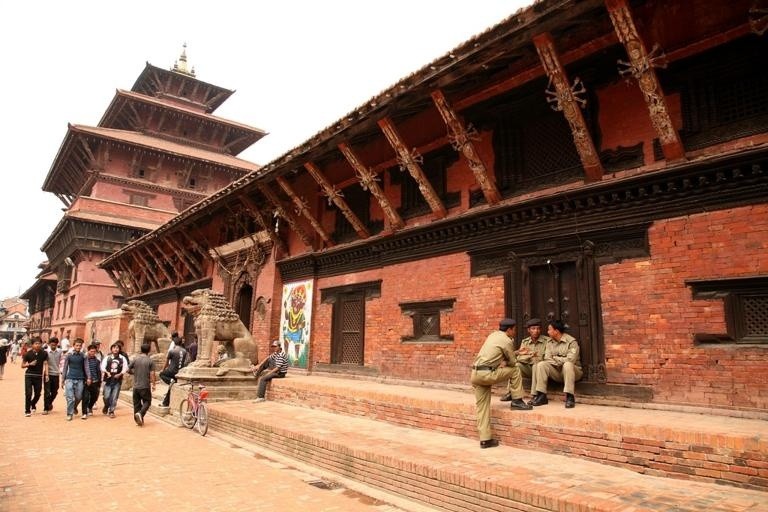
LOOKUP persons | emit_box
[252,340,288,403]
[212,345,231,368]
[471,318,533,448]
[531,319,584,408]
[0,330,198,426]
[500,317,549,404]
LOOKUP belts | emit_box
[472,366,493,369]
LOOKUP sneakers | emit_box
[25,405,52,416]
[135,413,143,425]
[67,406,115,420]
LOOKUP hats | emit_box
[499,320,516,326]
[271,340,280,346]
[0,338,8,346]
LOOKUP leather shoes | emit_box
[480,439,498,448]
[500,392,548,409]
[566,395,574,407]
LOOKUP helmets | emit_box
[528,319,541,326]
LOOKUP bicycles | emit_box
[178,379,211,437]
